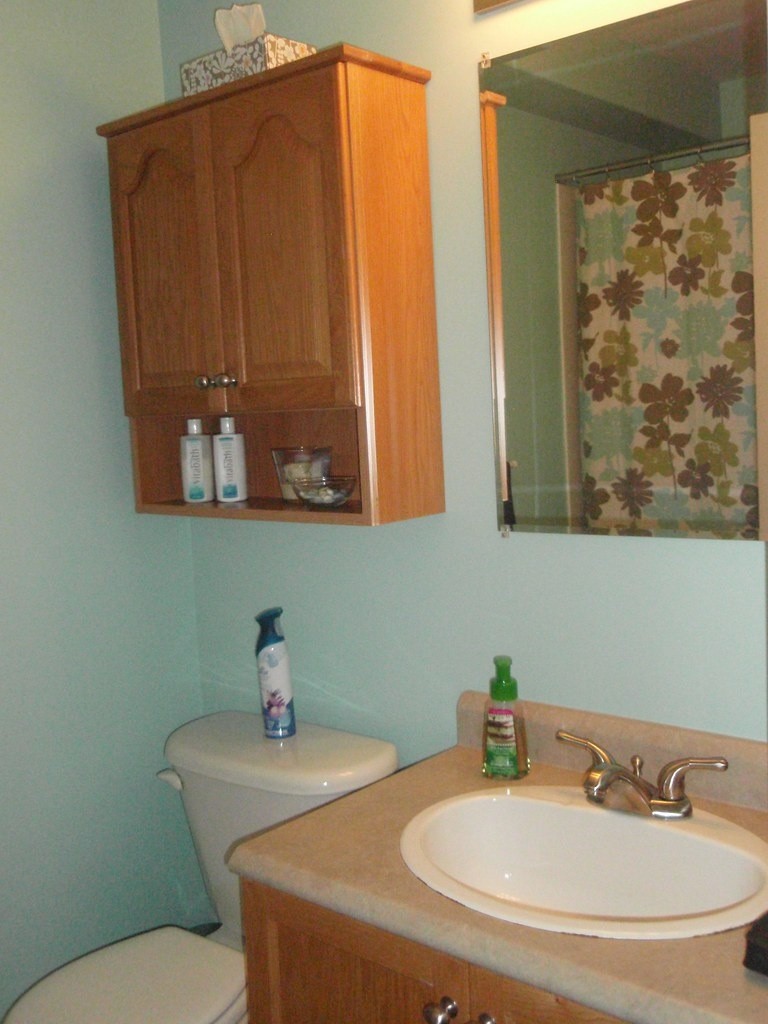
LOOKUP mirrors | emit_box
[478,1,767,539]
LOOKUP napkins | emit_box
[214,3,267,49]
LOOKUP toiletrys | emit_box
[212,416,249,503]
[477,653,535,780]
[178,416,218,504]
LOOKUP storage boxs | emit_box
[179,33,318,98]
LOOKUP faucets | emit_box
[582,752,663,821]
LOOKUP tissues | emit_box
[177,1,319,103]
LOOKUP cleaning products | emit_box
[252,606,296,740]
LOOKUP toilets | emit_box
[0,706,409,1024]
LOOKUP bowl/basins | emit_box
[293,476,356,506]
[270,445,334,503]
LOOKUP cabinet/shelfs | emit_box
[237,875,625,1024]
[93,42,448,527]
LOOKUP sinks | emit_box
[399,775,766,941]
